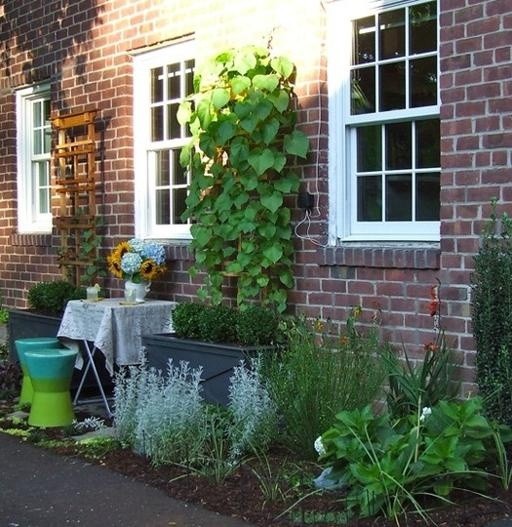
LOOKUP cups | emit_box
[124,288,137,302]
[87,287,99,303]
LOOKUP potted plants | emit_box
[0,308,10,344]
[142,303,287,407]
[9,280,112,390]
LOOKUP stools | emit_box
[14,338,78,427]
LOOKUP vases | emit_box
[125,281,151,303]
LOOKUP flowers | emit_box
[107,238,168,284]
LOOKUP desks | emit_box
[57,297,180,419]
